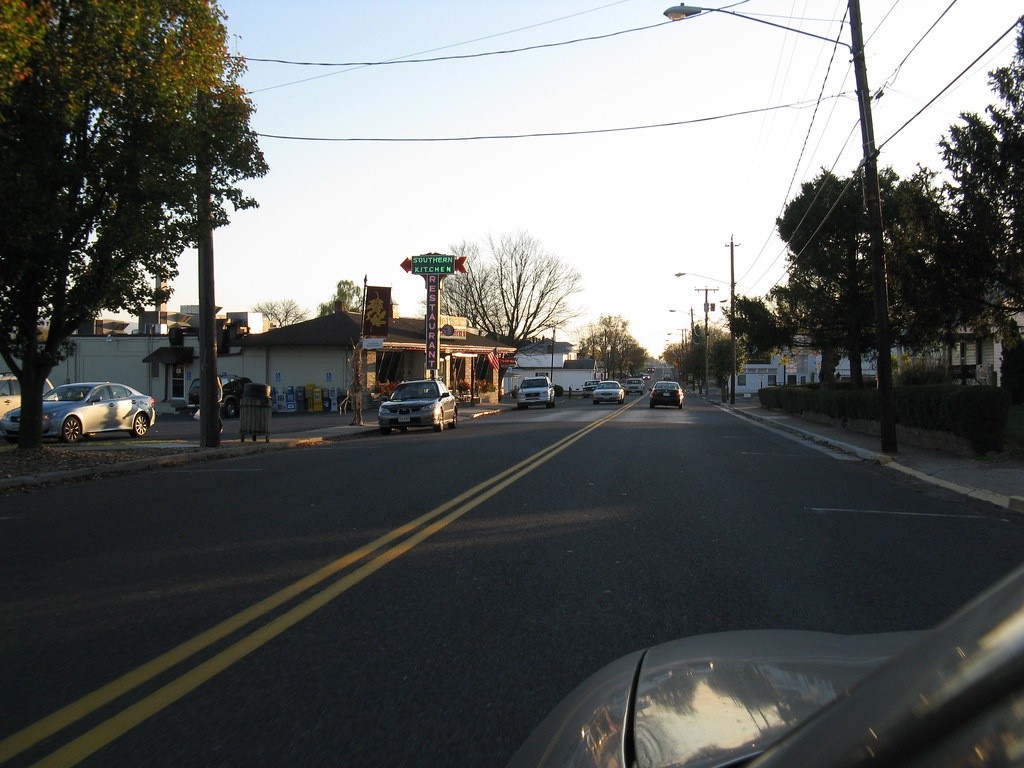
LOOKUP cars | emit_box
[647,367,655,373]
[626,378,645,395]
[664,374,671,380]
[591,380,626,404]
[0,381,155,444]
[649,381,684,408]
[581,380,601,398]
[640,374,650,380]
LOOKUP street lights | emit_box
[668,308,695,390]
[672,235,737,407]
[667,332,684,377]
[659,3,901,456]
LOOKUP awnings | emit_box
[143,346,194,364]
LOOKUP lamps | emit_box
[106,334,119,344]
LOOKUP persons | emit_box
[216,377,224,434]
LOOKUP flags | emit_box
[486,348,500,369]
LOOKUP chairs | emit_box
[409,391,418,398]
[527,382,533,387]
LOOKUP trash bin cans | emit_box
[975,363,997,386]
[239,383,274,442]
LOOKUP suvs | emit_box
[378,378,457,436]
[515,375,556,409]
[0,371,59,421]
[187,373,255,417]
